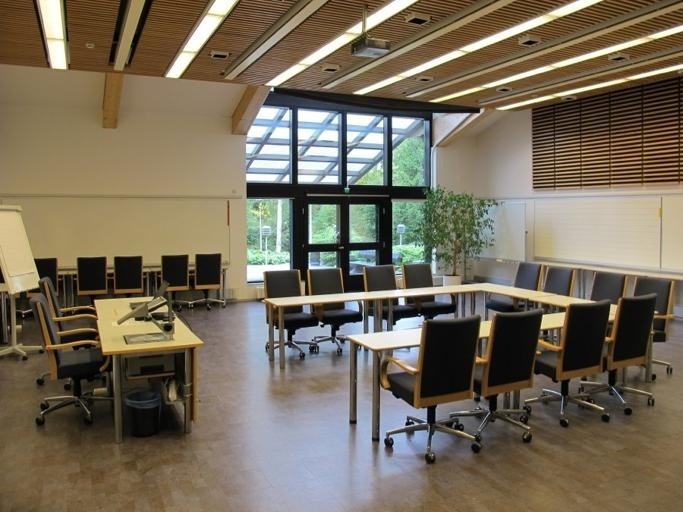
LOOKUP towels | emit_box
[125,389,161,439]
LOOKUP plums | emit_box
[350,38,391,59]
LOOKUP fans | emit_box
[533,190,683,276]
[465,203,527,262]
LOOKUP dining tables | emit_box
[412,187,507,286]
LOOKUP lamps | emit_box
[541,265,577,340]
[36,277,99,391]
[402,261,457,321]
[34,258,61,296]
[75,255,109,296]
[160,253,193,311]
[578,293,659,414]
[262,269,320,359]
[525,299,612,428]
[30,293,114,426]
[485,260,544,313]
[113,255,145,295]
[189,253,226,310]
[361,263,419,333]
[631,277,677,381]
[449,309,533,444]
[589,270,628,307]
[383,315,482,464]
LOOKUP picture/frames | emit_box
[57,265,233,315]
[94,296,204,444]
[264,281,659,442]
[306,267,363,355]
